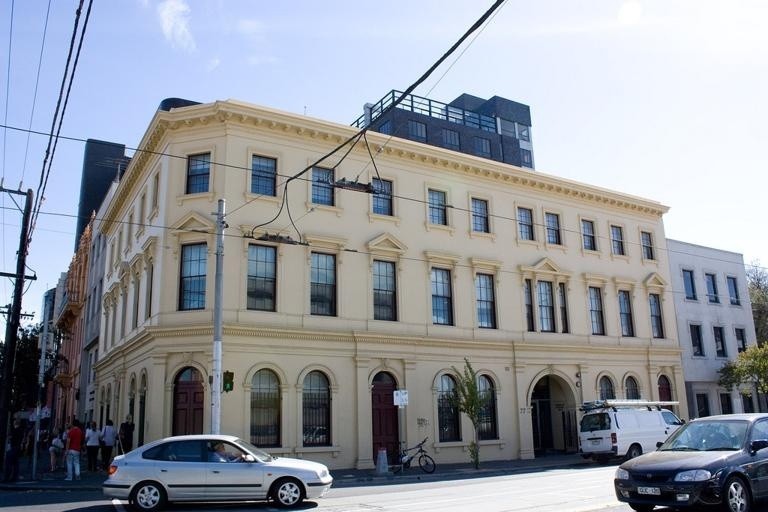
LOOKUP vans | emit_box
[573,397,681,462]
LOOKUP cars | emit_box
[303,425,327,444]
[100,433,335,509]
[613,413,767,508]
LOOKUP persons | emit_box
[211,441,246,464]
[2,413,136,481]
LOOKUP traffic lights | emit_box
[223,370,234,390]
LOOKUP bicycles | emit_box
[385,437,437,475]
